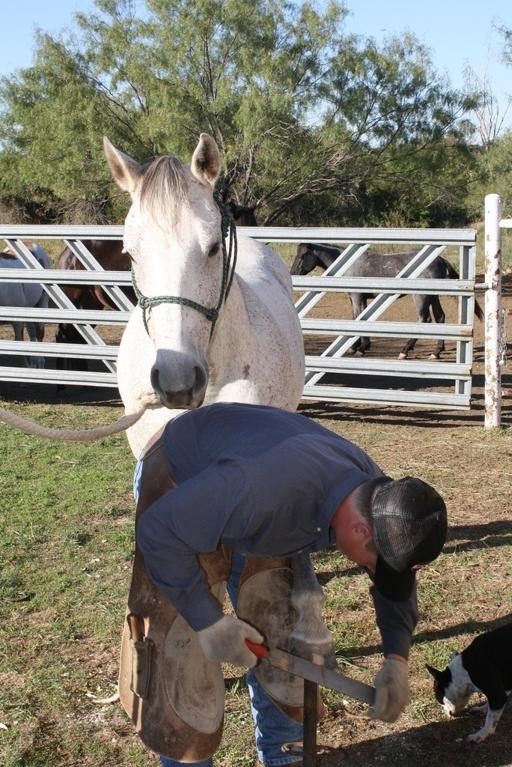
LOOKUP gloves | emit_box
[368,651,411,726]
[196,614,265,669]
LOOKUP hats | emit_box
[371,475,448,603]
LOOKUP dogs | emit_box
[420,618,512,742]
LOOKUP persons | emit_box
[114,399,449,765]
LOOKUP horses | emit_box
[0,242,54,386]
[52,199,264,398]
[93,126,310,466]
[289,239,484,360]
